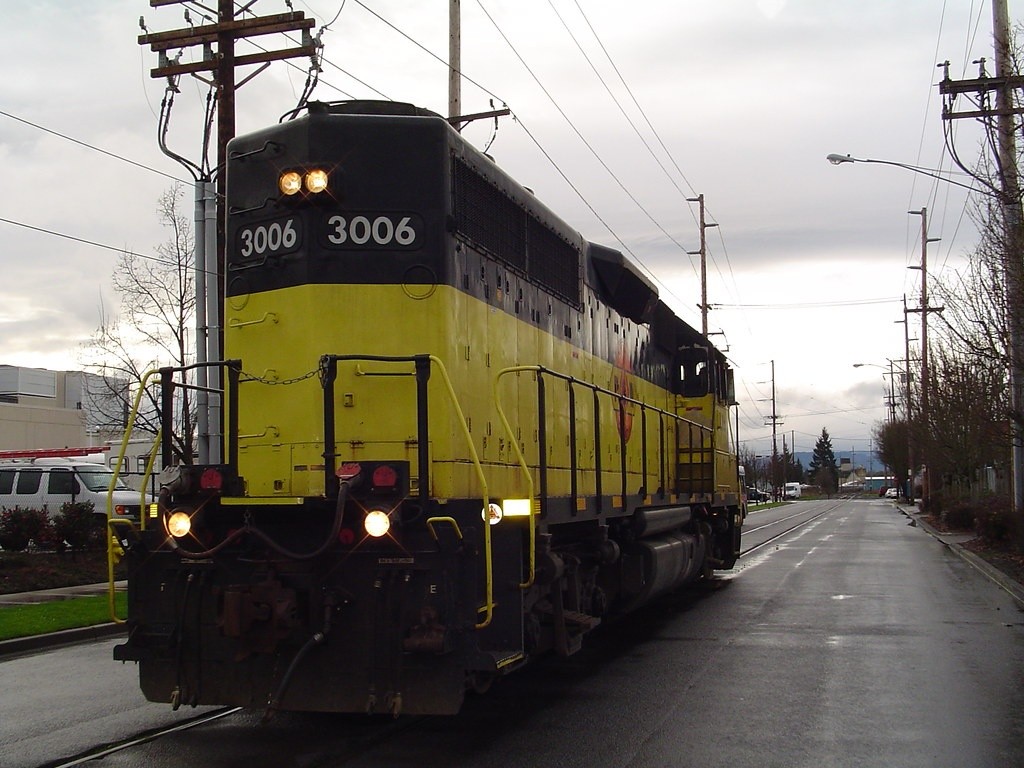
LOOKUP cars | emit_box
[879,485,904,500]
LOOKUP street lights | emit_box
[822,153,1023,518]
[850,362,915,507]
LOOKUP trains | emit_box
[106,101,747,723]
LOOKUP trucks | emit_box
[781,481,802,500]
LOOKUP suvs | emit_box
[1,457,161,548]
[747,488,771,503]
